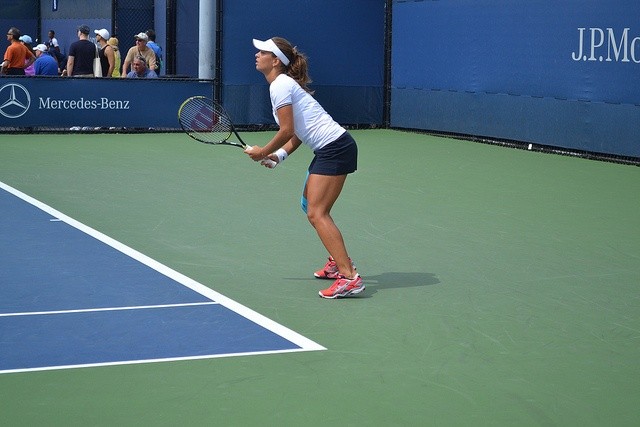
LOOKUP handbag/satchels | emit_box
[93,43,102,77]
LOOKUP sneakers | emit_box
[318,273,365,299]
[314,256,357,278]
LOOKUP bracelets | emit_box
[273,148,288,163]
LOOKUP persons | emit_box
[48,30,60,53]
[126,56,158,79]
[67,24,98,78]
[43,41,66,75]
[18,35,36,76]
[122,32,156,78]
[106,37,121,78]
[33,43,58,76]
[145,30,163,78]
[243,37,365,300]
[2,28,37,75]
[94,28,115,78]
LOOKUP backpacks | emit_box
[149,42,160,74]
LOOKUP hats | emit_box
[94,28,110,40]
[134,32,148,41]
[19,35,32,44]
[253,38,291,66]
[33,44,48,52]
[107,37,119,46]
